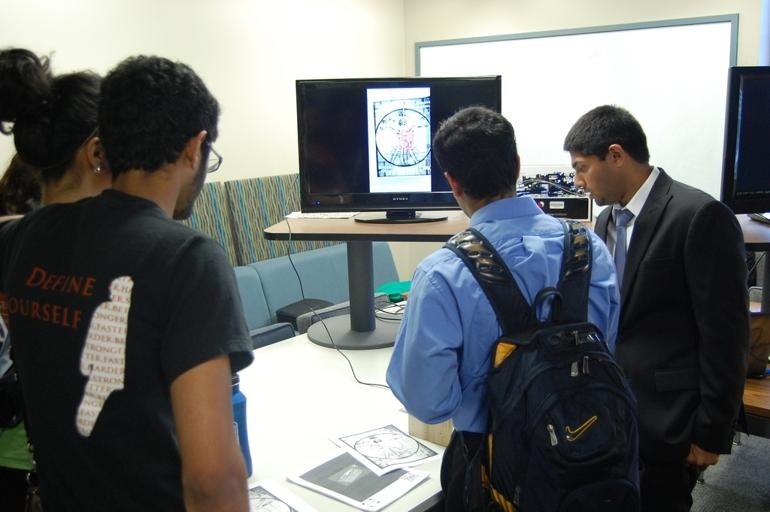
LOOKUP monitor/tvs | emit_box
[721,67,770,215]
[295,76,501,223]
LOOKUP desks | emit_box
[727,213,770,316]
[231,302,484,511]
[265,210,470,351]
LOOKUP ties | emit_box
[612,209,634,287]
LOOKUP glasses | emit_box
[203,141,223,174]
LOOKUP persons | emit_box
[382,104,622,509]
[0,47,121,508]
[0,53,255,512]
[558,106,751,512]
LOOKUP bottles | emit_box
[228,372,253,478]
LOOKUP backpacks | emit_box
[437,218,642,512]
